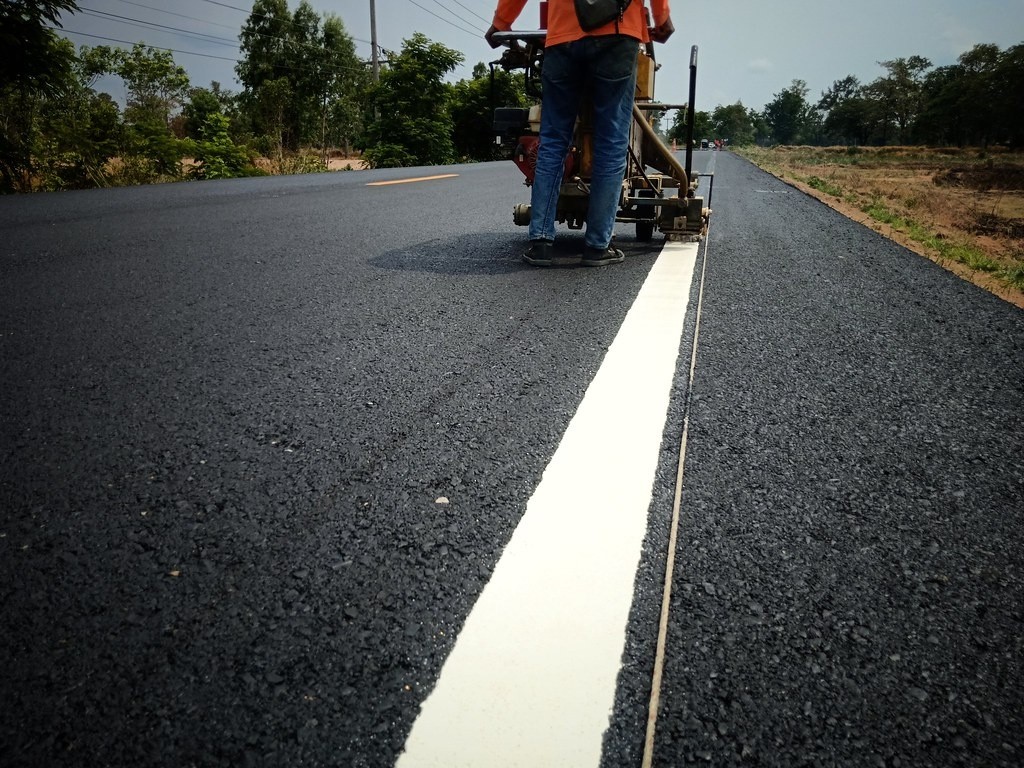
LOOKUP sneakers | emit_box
[523,240,553,266]
[582,240,625,266]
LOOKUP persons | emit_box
[485,0,675,266]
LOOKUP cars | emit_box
[691,138,715,149]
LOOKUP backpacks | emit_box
[574,0,632,32]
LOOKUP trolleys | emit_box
[483,30,713,241]
